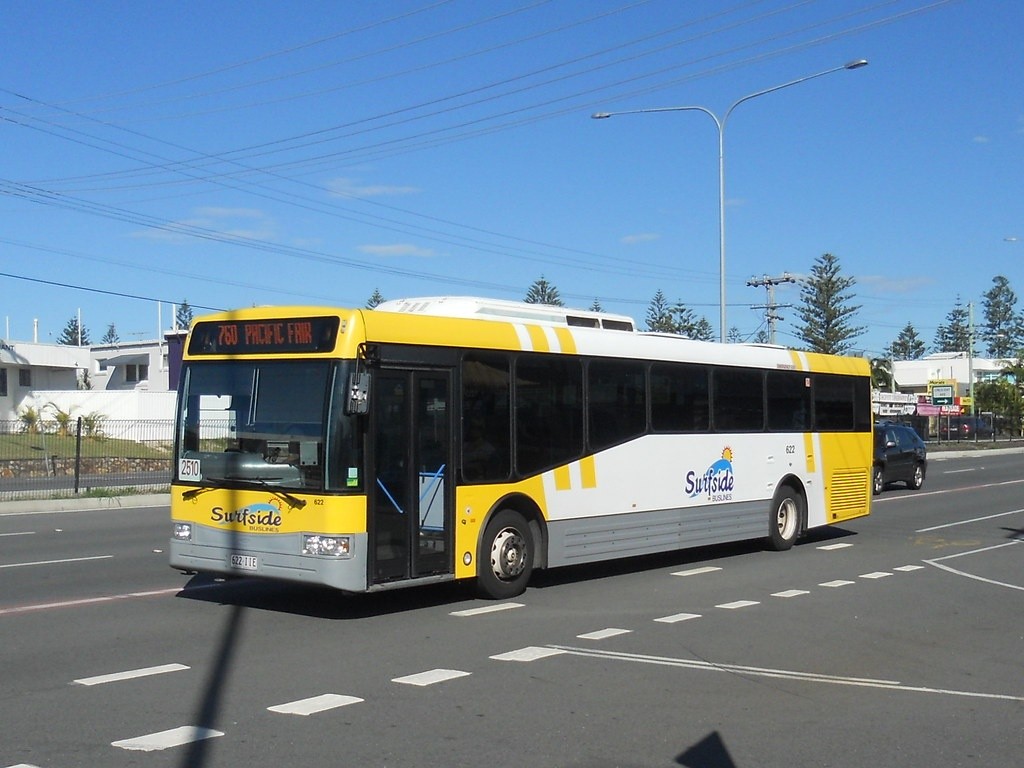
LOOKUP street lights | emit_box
[591,57,869,342]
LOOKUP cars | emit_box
[871,423,927,496]
[940,417,994,441]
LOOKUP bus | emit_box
[169,293,874,600]
